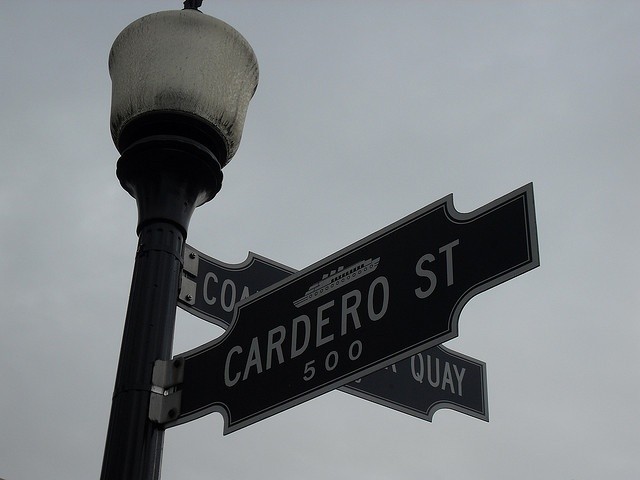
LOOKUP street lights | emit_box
[94,1,260,479]
